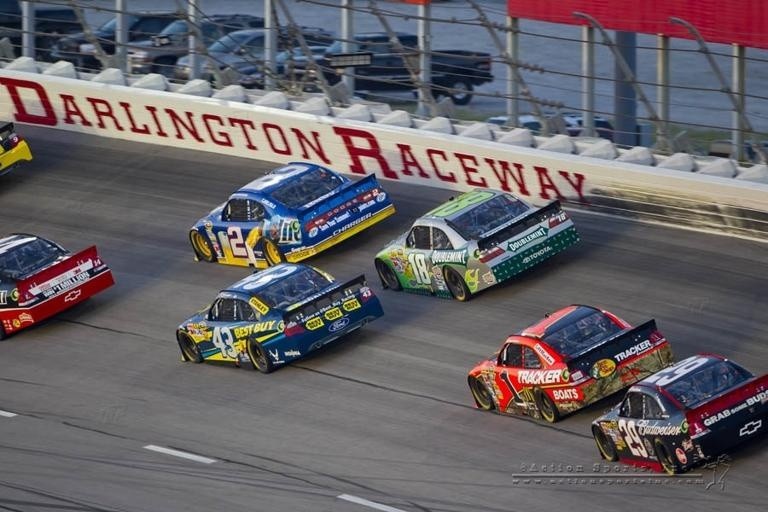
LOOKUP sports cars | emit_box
[0,231,115,342]
[0,121,33,179]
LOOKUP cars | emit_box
[486,112,612,140]
